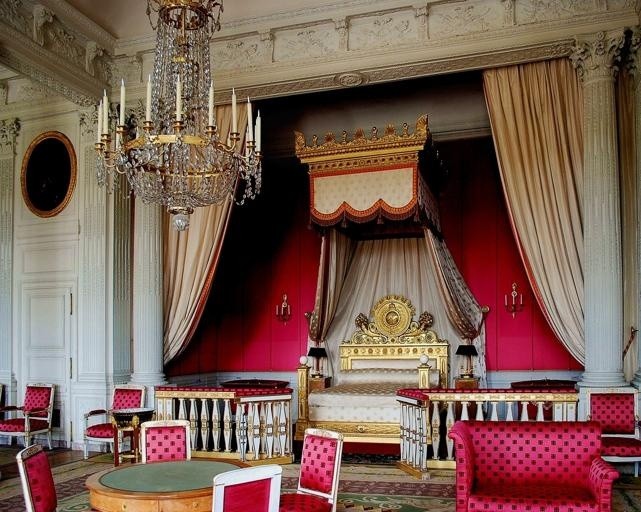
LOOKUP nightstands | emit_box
[307,374,332,392]
[454,376,481,419]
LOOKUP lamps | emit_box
[88,0,270,233]
[305,342,329,378]
[455,344,479,378]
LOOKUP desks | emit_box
[218,376,291,389]
[84,457,255,512]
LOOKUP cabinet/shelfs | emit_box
[511,378,580,419]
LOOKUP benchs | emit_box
[446,419,621,512]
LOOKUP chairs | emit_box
[581,386,640,479]
[0,381,60,457]
[279,427,343,512]
[13,442,58,512]
[210,463,285,512]
[82,384,149,464]
[138,419,192,466]
[0,381,6,420]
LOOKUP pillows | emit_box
[337,364,442,386]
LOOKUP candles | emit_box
[511,293,515,304]
[286,304,290,314]
[281,305,283,314]
[503,294,508,305]
[520,293,522,303]
[275,303,278,315]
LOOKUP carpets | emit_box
[0,441,71,467]
[0,446,640,512]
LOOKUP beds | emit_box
[288,112,496,464]
